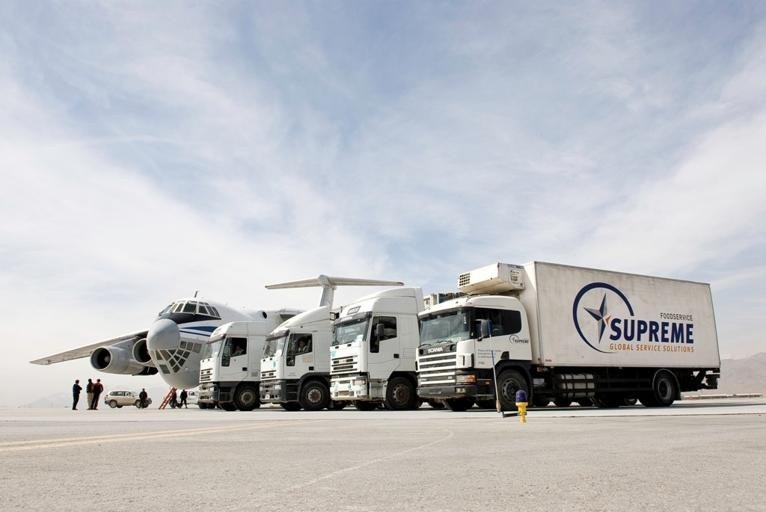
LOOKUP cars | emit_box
[176,390,198,405]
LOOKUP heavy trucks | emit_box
[329,287,468,411]
[415,260,722,413]
[197,307,308,411]
[257,304,352,410]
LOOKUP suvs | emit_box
[103,389,152,410]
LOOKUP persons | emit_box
[86,378,103,410]
[295,340,309,354]
[179,388,188,408]
[86,378,99,410]
[172,391,180,409]
[231,345,245,357]
[72,379,82,410]
[138,387,147,408]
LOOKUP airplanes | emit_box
[28,273,405,411]
